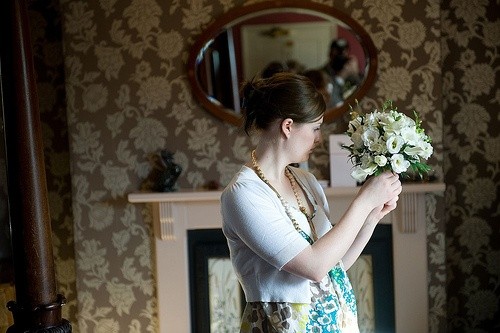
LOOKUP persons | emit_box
[261,26,363,106]
[219,72,403,333]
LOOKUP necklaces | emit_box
[251,148,318,242]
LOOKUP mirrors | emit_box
[187,0,378,129]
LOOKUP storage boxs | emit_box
[329,134,357,188]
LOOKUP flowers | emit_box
[341,98,434,183]
